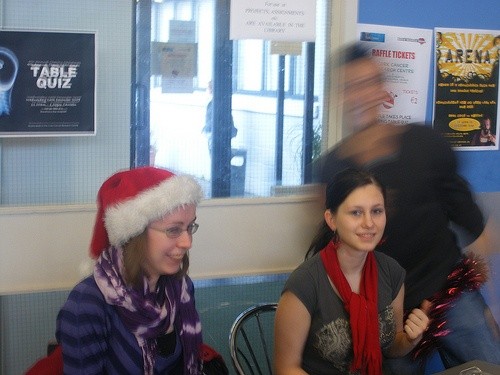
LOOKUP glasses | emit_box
[148,221,199,238]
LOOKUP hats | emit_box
[88,166,203,260]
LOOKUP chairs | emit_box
[228,303,278,375]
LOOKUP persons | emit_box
[55,166,203,375]
[274,171,431,375]
[314,39,500,375]
